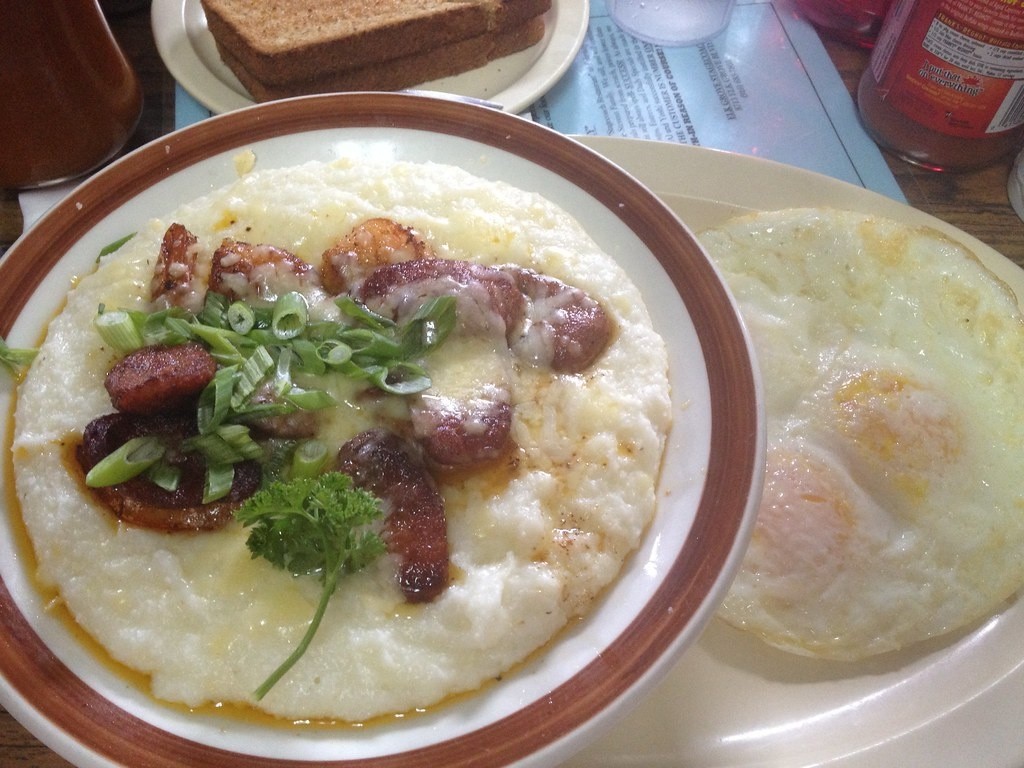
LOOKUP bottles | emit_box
[855,0,1024,173]
[0,1,145,191]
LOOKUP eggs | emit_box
[689,202,1024,658]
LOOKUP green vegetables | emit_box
[233,468,387,698]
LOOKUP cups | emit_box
[603,0,737,48]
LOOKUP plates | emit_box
[150,0,591,112]
[559,134,1024,768]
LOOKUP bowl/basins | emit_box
[1,90,764,767]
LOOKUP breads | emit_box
[199,0,552,104]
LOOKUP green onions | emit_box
[1,235,453,508]
